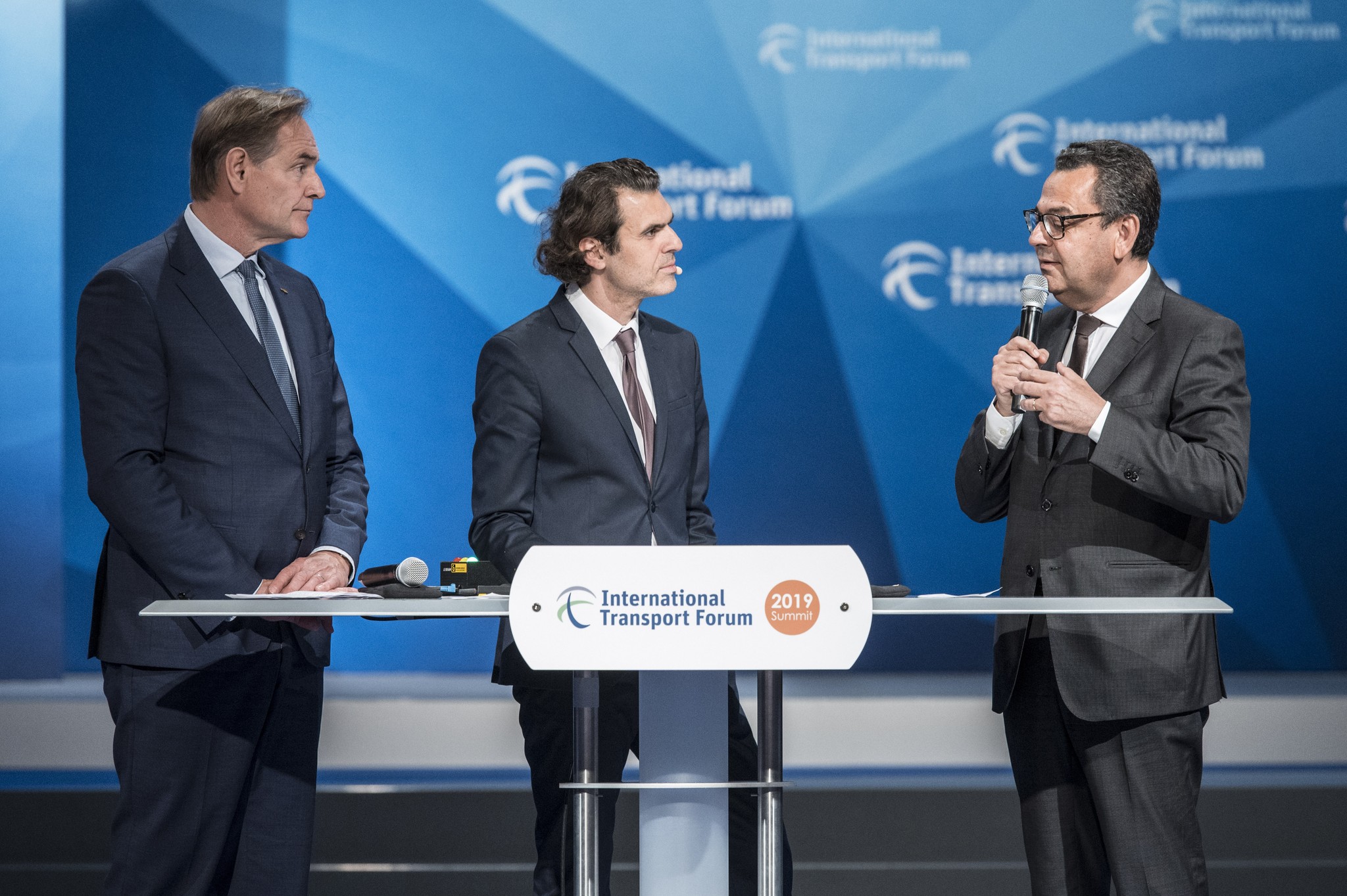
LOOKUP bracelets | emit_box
[338,553,351,563]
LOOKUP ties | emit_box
[1066,314,1103,380]
[613,328,659,485]
[234,260,302,444]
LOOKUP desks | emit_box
[140,596,1233,896]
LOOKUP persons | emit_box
[468,158,795,896]
[954,140,1250,896]
[75,87,369,896]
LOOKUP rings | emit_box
[1033,398,1038,412]
[316,574,324,579]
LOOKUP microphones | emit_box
[676,266,682,275]
[358,556,428,588]
[1011,274,1049,414]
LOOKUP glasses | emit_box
[1024,207,1112,240]
